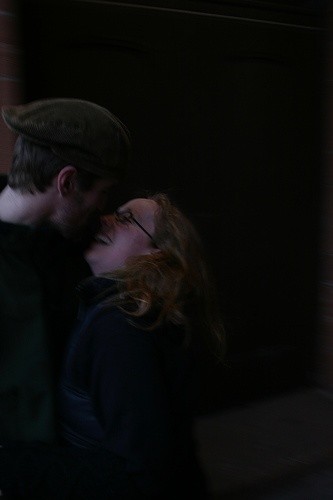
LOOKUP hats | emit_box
[1,99,138,180]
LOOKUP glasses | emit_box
[110,206,159,249]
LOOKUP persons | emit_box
[55,191,228,500]
[0,98,132,499]
